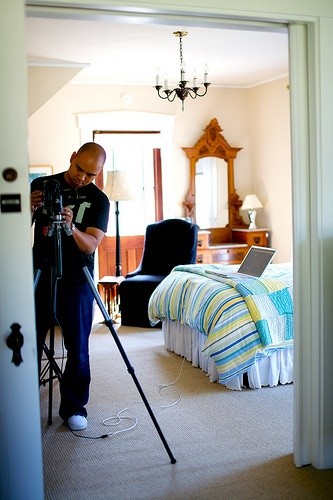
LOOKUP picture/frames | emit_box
[29,163,54,186]
[236,245,278,279]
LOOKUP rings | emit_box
[67,211,70,215]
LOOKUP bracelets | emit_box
[71,222,75,231]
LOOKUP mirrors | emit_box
[194,154,230,229]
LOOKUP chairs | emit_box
[120,219,198,329]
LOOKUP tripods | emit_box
[34,212,177,464]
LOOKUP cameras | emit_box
[38,180,78,209]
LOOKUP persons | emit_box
[30,142,110,430]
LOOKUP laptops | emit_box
[205,246,277,278]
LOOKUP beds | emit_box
[148,261,293,391]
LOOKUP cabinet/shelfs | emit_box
[198,229,268,264]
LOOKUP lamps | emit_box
[154,31,211,110]
[241,194,263,230]
[101,171,136,277]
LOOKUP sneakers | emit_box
[68,414,87,430]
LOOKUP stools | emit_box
[98,276,124,324]
